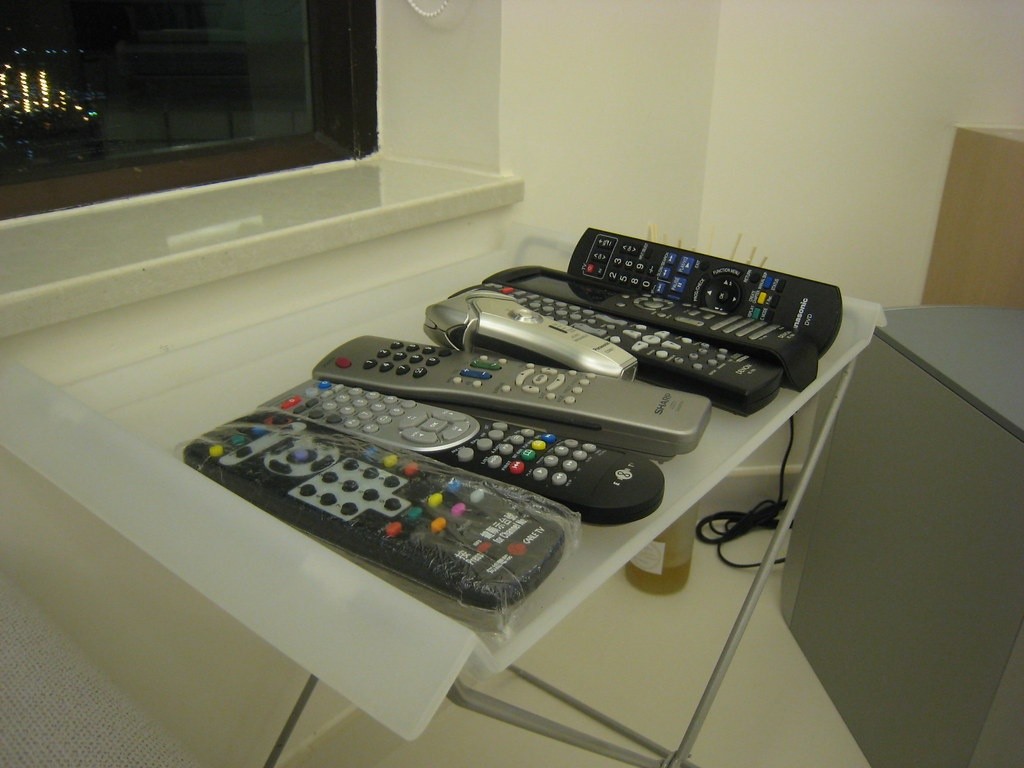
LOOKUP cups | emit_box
[625,502,699,595]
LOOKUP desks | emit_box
[0,223,888,768]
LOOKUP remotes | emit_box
[182,226,846,613]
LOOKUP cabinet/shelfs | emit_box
[776,329,1024,768]
